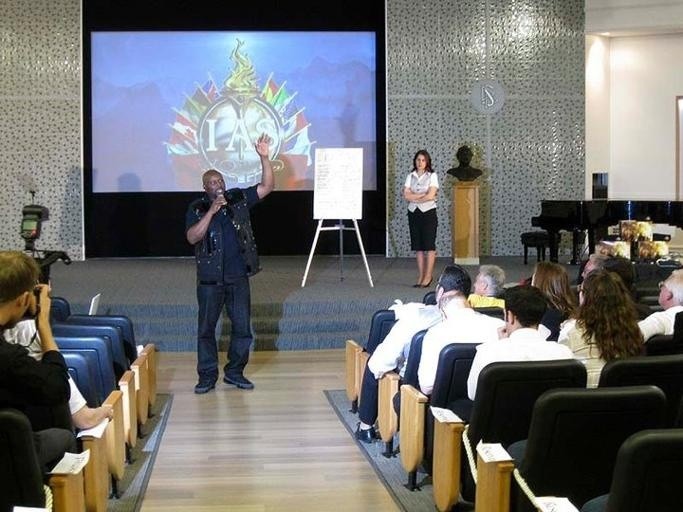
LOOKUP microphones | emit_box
[215,188,228,216]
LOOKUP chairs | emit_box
[344,283,683,511]
[0,296,158,512]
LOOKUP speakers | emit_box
[591,173,607,198]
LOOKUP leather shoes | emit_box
[355,427,375,443]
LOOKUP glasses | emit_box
[577,284,584,293]
[658,281,673,300]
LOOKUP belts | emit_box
[200,280,235,286]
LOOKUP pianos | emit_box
[531,200,683,264]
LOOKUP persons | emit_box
[1,250,79,486]
[472,264,508,297]
[4,318,115,429]
[403,149,439,289]
[183,132,274,394]
[636,268,683,342]
[387,291,436,311]
[392,289,509,419]
[529,261,582,341]
[466,284,579,401]
[559,269,644,389]
[445,145,482,182]
[352,264,472,444]
[597,256,654,321]
[581,252,612,281]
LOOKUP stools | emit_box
[520,229,554,266]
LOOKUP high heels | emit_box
[419,276,433,288]
[413,275,425,287]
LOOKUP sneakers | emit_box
[224,374,254,389]
[195,372,219,393]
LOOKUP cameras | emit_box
[33,288,41,318]
